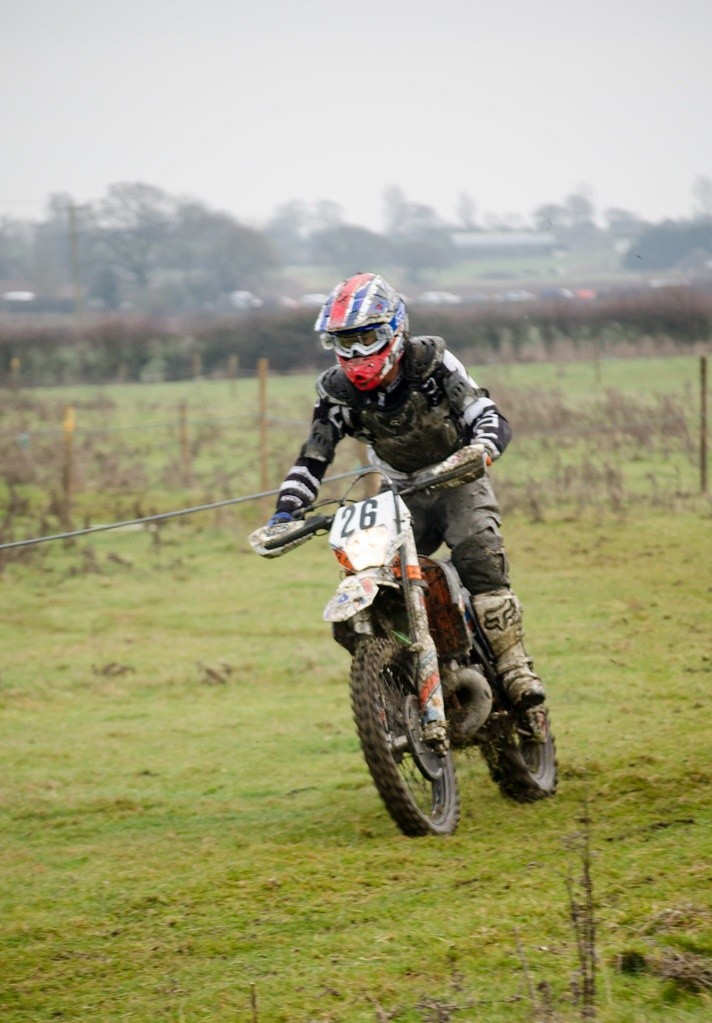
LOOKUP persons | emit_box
[269,272,545,710]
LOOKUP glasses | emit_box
[333,327,387,358]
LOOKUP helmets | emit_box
[313,273,409,392]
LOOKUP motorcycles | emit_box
[247,438,560,839]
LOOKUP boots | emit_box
[474,590,545,713]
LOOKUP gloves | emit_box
[265,510,295,526]
